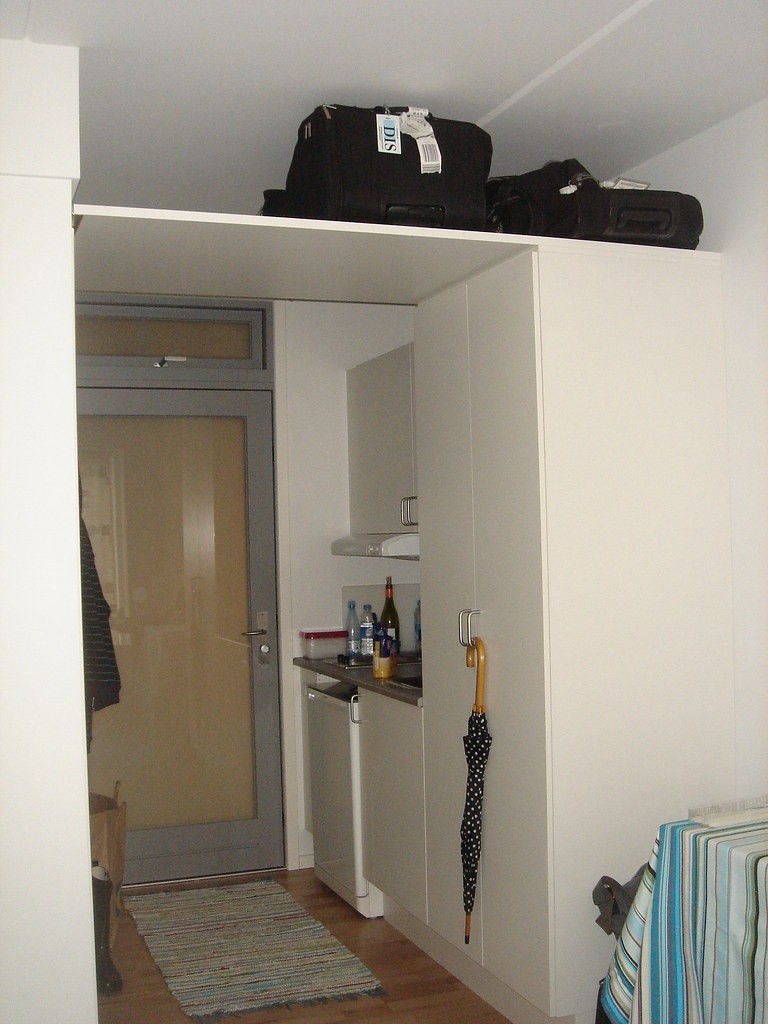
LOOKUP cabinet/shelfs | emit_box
[346,341,417,534]
[350,685,428,926]
[423,245,729,1024]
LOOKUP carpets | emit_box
[119,878,388,1022]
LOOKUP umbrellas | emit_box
[459,637,493,944]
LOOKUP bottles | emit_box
[358,604,374,656]
[345,601,362,658]
[413,599,421,652]
[379,576,400,658]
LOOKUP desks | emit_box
[596,806,768,1024]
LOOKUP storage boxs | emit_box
[88,792,127,948]
